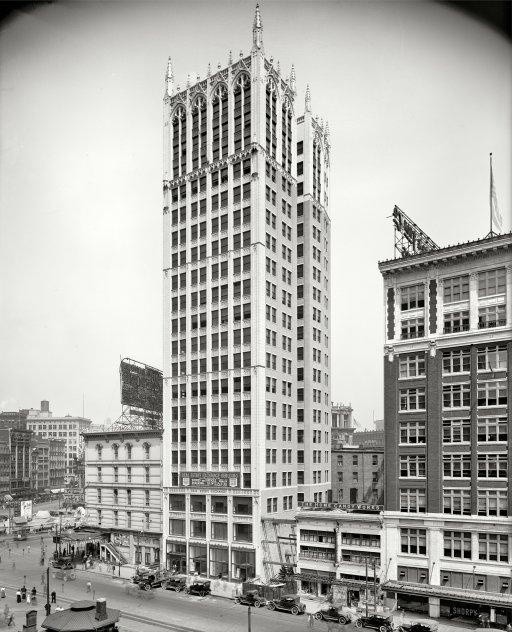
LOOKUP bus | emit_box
[133,567,211,597]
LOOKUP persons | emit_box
[1,552,94,626]
[308,612,315,629]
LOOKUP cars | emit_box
[398,619,439,632]
[52,557,74,570]
[234,590,268,608]
[356,612,394,632]
[314,605,351,625]
[267,594,306,615]
